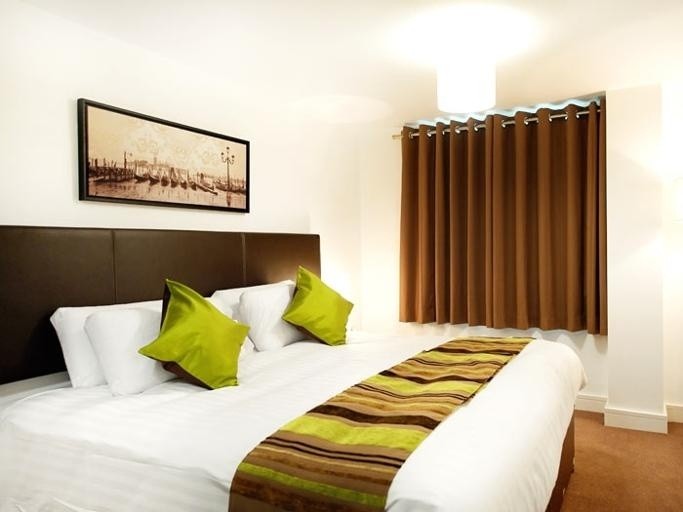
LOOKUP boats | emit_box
[134,170,151,182]
[177,169,186,189]
[160,169,168,187]
[170,167,177,186]
[216,178,245,193]
[149,169,160,184]
[187,171,196,191]
[200,173,214,191]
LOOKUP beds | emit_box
[0,230,586,512]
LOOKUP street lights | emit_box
[221,146,234,206]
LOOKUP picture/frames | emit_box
[77,97,251,214]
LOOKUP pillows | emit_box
[211,279,296,321]
[138,279,252,390]
[237,284,310,353]
[83,307,256,397]
[47,298,164,391]
[281,266,354,346]
